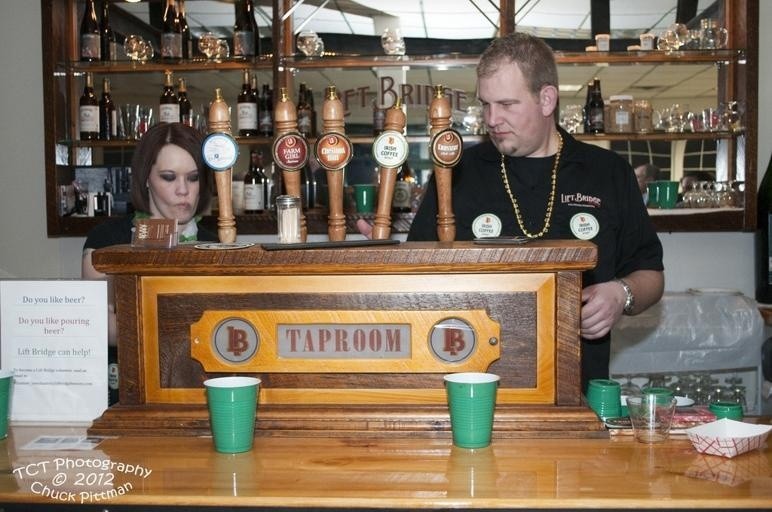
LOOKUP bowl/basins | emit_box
[686,418,772,458]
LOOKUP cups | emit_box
[700,18,728,49]
[646,181,659,209]
[634,99,661,133]
[688,31,699,49]
[642,386,674,405]
[443,372,501,448]
[657,180,679,209]
[661,106,671,132]
[670,104,689,132]
[710,402,743,421]
[0,373,12,440]
[138,106,153,137]
[120,104,139,137]
[588,379,622,420]
[354,184,377,214]
[722,102,740,131]
[627,395,676,444]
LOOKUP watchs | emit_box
[616,278,635,313]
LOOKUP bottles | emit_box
[81,0,100,62]
[258,152,268,209]
[308,89,317,135]
[303,164,310,211]
[590,77,606,134]
[100,78,117,138]
[78,72,100,141]
[178,0,192,59]
[610,95,634,133]
[307,166,317,209]
[237,68,259,136]
[100,0,117,62]
[299,76,312,138]
[251,71,260,96]
[161,71,183,123]
[179,74,196,122]
[243,146,262,214]
[161,1,182,60]
[270,162,282,211]
[248,1,260,56]
[393,160,411,213]
[235,0,256,57]
[277,197,301,244]
[582,83,592,133]
[604,103,611,133]
[260,81,273,138]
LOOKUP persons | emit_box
[81,122,222,403]
[407,33,665,396]
[675,173,713,201]
[632,164,659,205]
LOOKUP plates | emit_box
[677,396,694,406]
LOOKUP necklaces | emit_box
[501,131,564,238]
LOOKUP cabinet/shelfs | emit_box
[40,0,759,240]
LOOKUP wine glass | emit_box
[715,180,736,207]
[706,182,720,207]
[384,39,405,56]
[692,181,711,207]
[318,38,324,58]
[666,23,689,50]
[142,41,153,62]
[682,182,698,207]
[204,377,260,454]
[219,40,230,58]
[124,35,145,60]
[199,33,218,58]
[381,27,400,55]
[296,32,318,57]
[656,32,666,50]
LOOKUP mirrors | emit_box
[71,0,725,57]
[71,66,721,133]
[72,139,732,204]
